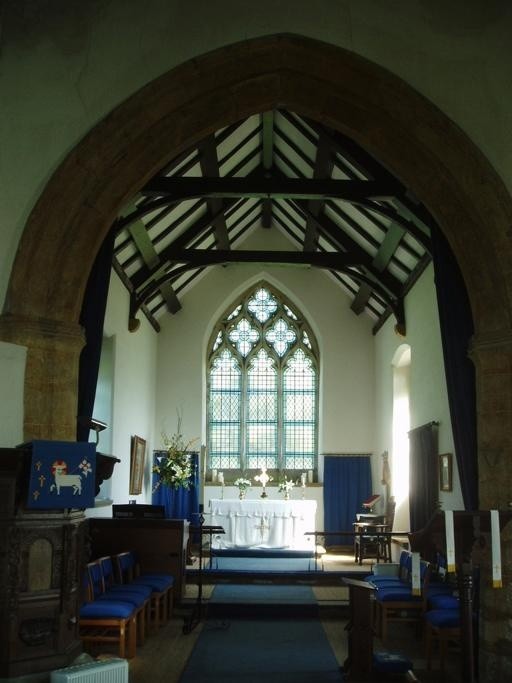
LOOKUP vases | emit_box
[284,492,289,500]
[239,490,245,500]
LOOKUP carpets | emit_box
[179,555,344,683]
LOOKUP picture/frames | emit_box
[438,453,452,492]
[130,435,146,494]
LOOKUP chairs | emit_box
[79,551,175,659]
[363,550,480,671]
[352,501,396,565]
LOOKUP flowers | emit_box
[278,479,294,493]
[234,478,251,490]
[152,405,201,492]
